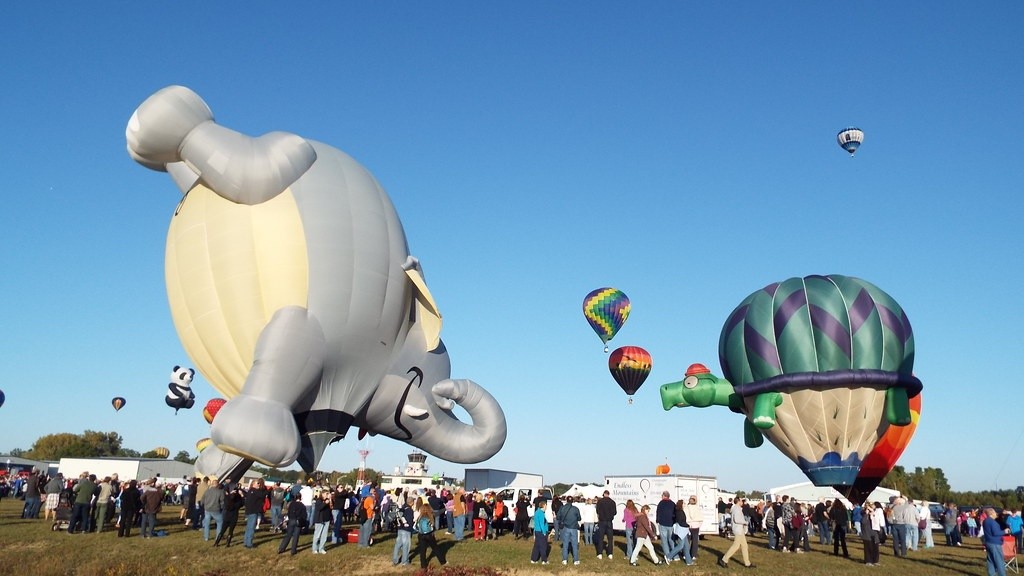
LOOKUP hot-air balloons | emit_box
[582,287,631,354]
[124,87,506,488]
[656,463,670,475]
[165,365,196,416]
[110,396,126,412]
[608,346,653,404]
[836,126,864,158]
[660,275,922,535]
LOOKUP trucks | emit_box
[603,473,719,540]
[478,486,598,532]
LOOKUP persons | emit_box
[556,496,581,566]
[531,502,551,566]
[657,492,680,561]
[716,494,934,564]
[622,500,638,559]
[0,467,509,547]
[245,479,265,549]
[594,490,617,559]
[683,495,703,560]
[982,508,1011,576]
[392,497,415,566]
[277,491,308,556]
[416,502,448,569]
[829,498,849,556]
[200,480,225,542]
[552,492,604,545]
[939,502,1024,550]
[662,499,697,566]
[630,505,665,566]
[718,496,757,569]
[534,489,548,510]
[513,495,531,540]
[141,484,162,538]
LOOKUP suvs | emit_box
[958,506,1004,530]
[911,499,946,529]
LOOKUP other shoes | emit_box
[718,559,728,568]
[562,560,567,565]
[311,550,318,555]
[956,542,962,547]
[624,555,699,566]
[542,560,549,565]
[865,562,874,567]
[573,561,580,565]
[597,554,603,559]
[795,548,804,553]
[531,560,538,564]
[873,563,880,566]
[607,554,613,560]
[782,546,791,553]
[745,563,756,568]
[318,550,327,555]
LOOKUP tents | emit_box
[560,483,606,523]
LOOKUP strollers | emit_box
[51,493,81,531]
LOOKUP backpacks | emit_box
[387,506,410,528]
[886,505,896,524]
[419,515,432,534]
[355,501,370,526]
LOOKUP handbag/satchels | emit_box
[811,513,818,524]
[918,518,927,529]
[478,508,488,520]
[296,519,306,527]
[559,521,564,529]
